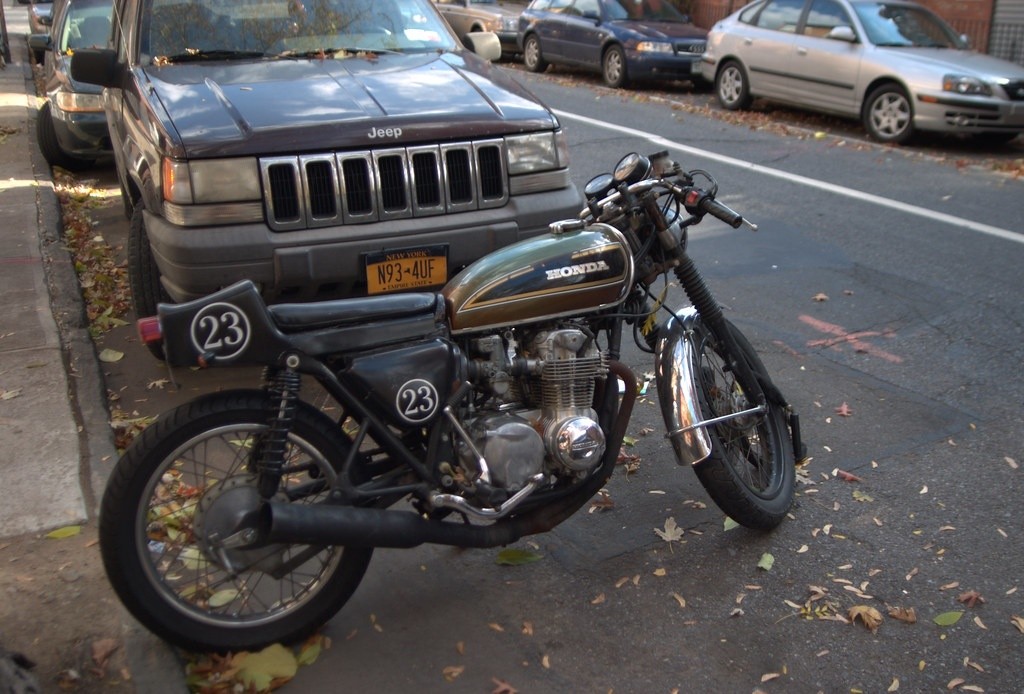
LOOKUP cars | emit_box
[402,0,529,63]
[515,0,715,93]
[17,0,117,177]
[699,0,1024,149]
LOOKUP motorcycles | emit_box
[99,128,809,659]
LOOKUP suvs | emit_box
[70,0,590,360]
